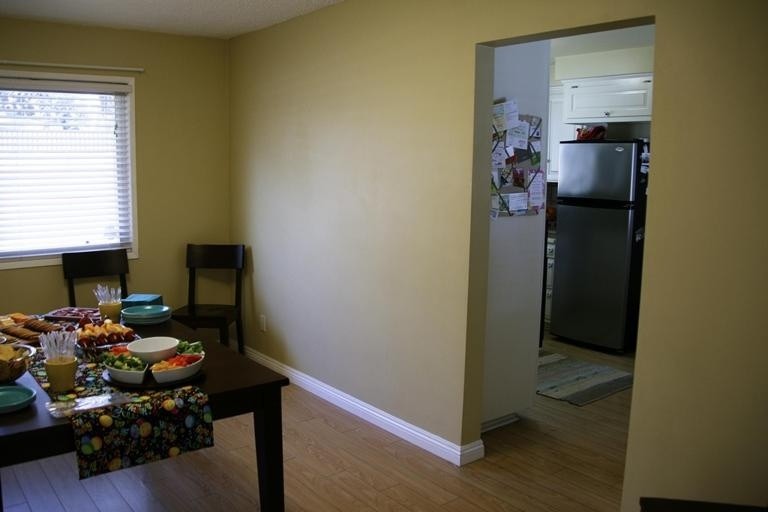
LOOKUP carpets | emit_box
[536,349,633,407]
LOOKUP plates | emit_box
[121,305,172,325]
[0,386,36,413]
[0,306,141,353]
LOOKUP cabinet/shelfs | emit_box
[544,237,557,323]
[547,73,651,183]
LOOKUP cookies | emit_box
[2,317,62,343]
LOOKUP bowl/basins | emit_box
[127,336,180,363]
[1,344,38,387]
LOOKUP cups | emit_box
[97,301,121,323]
[43,355,77,393]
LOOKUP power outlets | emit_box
[259,315,265,331]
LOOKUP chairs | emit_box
[63,249,130,307]
[172,243,245,356]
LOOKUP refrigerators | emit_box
[549,141,647,355]
